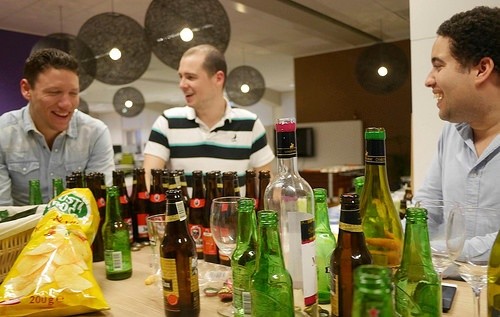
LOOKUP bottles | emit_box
[357,127,405,312]
[29,178,43,204]
[393,207,443,317]
[230,198,259,317]
[264,117,320,317]
[159,189,200,317]
[250,209,294,317]
[486,230,500,317]
[353,176,365,199]
[351,264,394,317]
[66,167,270,262]
[329,193,373,317]
[101,186,133,281]
[306,187,337,305]
[51,178,63,198]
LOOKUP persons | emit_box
[141,44,275,199]
[366,5,500,281]
[0,49,117,207]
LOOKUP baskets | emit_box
[0,211,46,284]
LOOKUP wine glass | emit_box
[415,199,466,317]
[210,196,257,317]
[146,213,167,288]
[445,207,500,317]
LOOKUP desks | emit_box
[92,235,488,317]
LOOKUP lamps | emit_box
[29,6,97,92]
[112,87,145,117]
[76,0,151,85]
[144,0,230,70]
[357,19,409,94]
[226,44,265,106]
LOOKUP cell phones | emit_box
[416,280,457,312]
